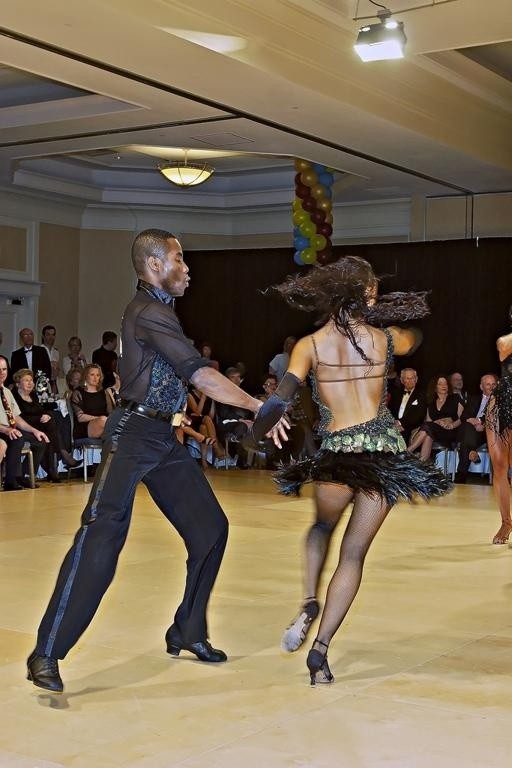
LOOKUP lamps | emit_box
[157,148,217,188]
[349,1,409,64]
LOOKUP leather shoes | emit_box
[4,478,39,491]
[165,623,226,662]
[27,651,63,691]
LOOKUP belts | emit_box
[123,400,172,421]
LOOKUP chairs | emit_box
[431,441,491,482]
[182,432,267,470]
[0,399,103,492]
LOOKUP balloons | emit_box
[292,159,333,267]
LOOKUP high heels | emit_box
[281,601,334,688]
[64,459,82,470]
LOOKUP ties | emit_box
[0,387,16,428]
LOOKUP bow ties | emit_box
[24,349,32,353]
[403,390,410,394]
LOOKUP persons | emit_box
[484,333,512,544]
[0,326,499,492]
[22,229,290,693]
[240,253,432,687]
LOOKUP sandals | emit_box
[203,437,216,445]
